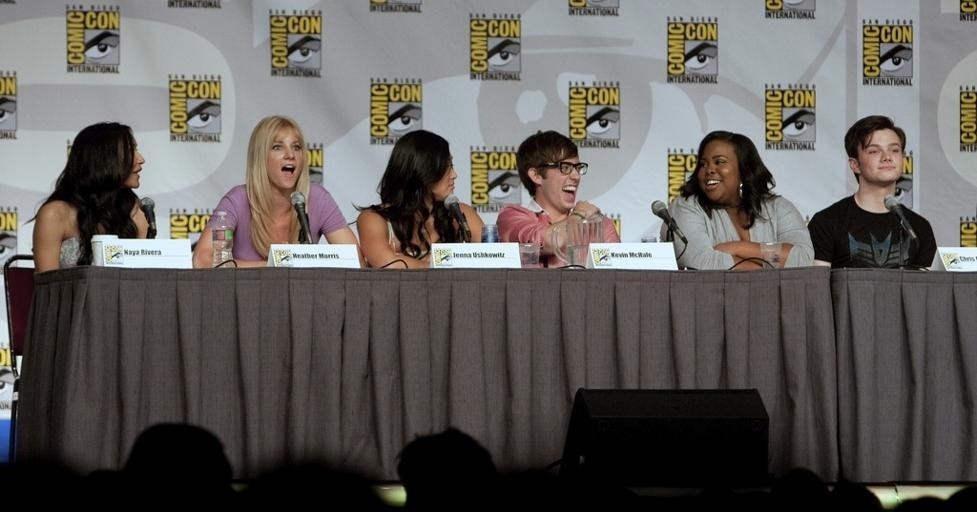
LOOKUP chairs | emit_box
[2,249,39,473]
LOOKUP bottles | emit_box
[212,211,234,268]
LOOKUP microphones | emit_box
[138,197,157,238]
[289,190,314,243]
[883,194,921,247]
[651,199,688,246]
[444,195,471,242]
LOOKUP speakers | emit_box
[557,387,769,489]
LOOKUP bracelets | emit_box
[570,211,585,220]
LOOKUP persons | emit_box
[22,122,150,272]
[0,419,976,512]
[191,115,365,269]
[356,128,486,269]
[496,130,620,268]
[658,130,817,271]
[807,116,937,272]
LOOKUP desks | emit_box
[7,259,845,492]
[823,266,975,489]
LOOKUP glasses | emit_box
[545,162,588,175]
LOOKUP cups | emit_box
[519,243,540,269]
[90,234,118,266]
[761,233,782,268]
[479,225,499,243]
[552,219,604,270]
[640,236,657,242]
